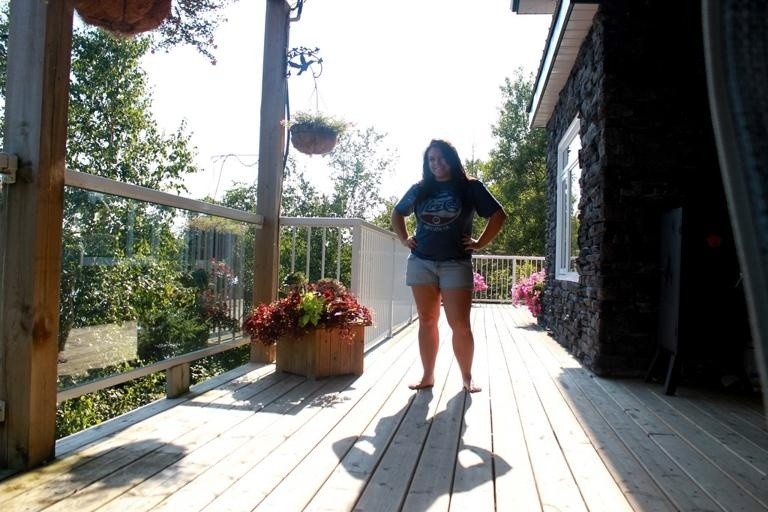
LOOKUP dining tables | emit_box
[82,238,241,363]
[239,272,376,379]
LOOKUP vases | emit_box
[471,265,486,293]
[146,0,239,66]
[508,264,548,316]
[278,106,356,144]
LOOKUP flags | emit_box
[536,308,546,325]
[291,129,338,155]
[70,0,177,38]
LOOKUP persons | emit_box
[392,140,506,393]
